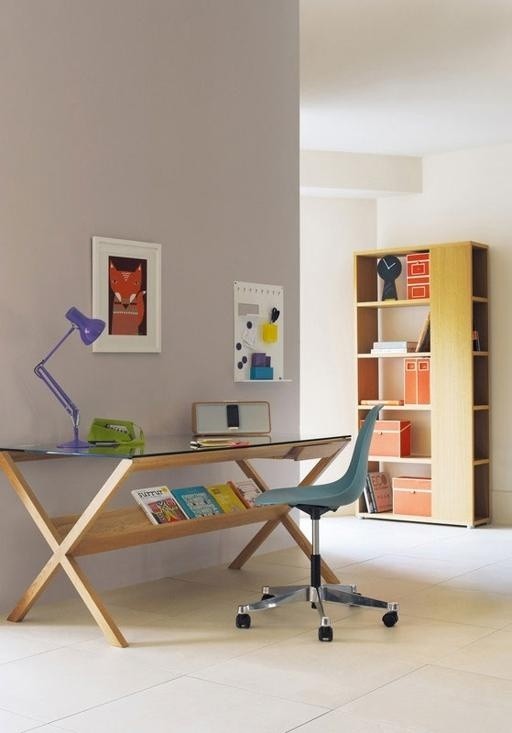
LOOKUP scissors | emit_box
[271,307,280,324]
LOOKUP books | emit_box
[196,437,233,446]
[228,478,270,507]
[130,485,190,525]
[373,341,417,347]
[171,485,225,519]
[371,346,416,353]
[361,398,404,405]
[206,483,247,512]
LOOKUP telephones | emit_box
[87,418,135,442]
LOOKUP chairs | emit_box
[233,404,400,642]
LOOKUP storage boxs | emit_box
[405,251,431,301]
[360,419,411,457]
[403,358,417,403]
[390,475,432,517]
[417,360,431,406]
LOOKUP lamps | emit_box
[34,306,106,450]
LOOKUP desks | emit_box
[1,430,353,649]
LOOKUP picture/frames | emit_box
[90,234,163,355]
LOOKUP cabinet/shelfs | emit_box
[353,240,492,531]
